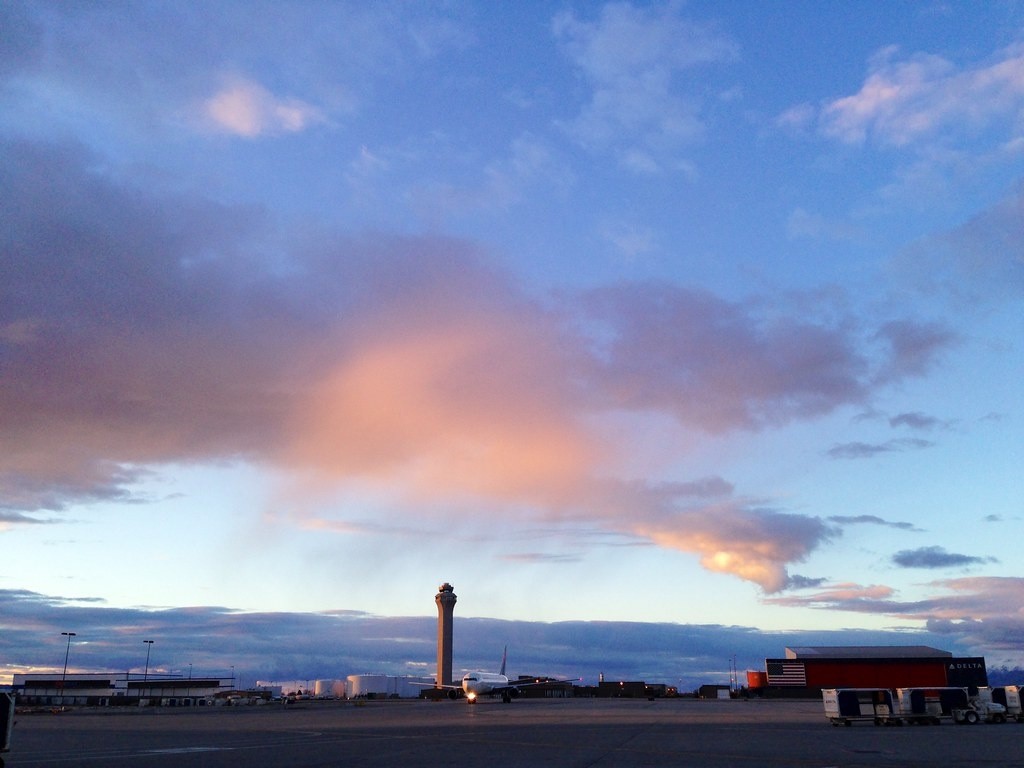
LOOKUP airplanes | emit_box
[408,645,580,704]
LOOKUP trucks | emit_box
[821,685,1024,727]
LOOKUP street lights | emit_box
[61,632,76,696]
[143,640,154,681]
